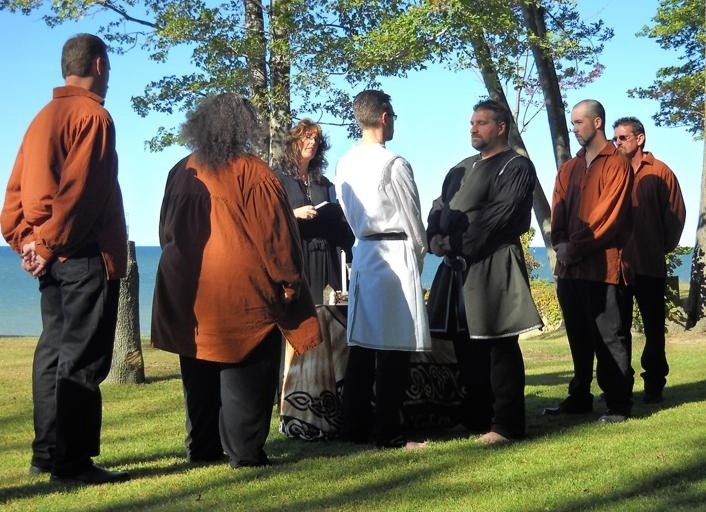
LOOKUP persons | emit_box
[335,91,428,450]
[271,119,353,443]
[426,99,545,445]
[599,116,686,404]
[0,34,131,487]
[542,100,634,424]
[150,93,324,469]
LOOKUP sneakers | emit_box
[404,442,425,450]
[639,393,663,404]
[474,432,510,448]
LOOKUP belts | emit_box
[441,254,471,272]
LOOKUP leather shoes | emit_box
[29,464,131,484]
[596,408,630,424]
[543,407,593,419]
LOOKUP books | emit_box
[301,199,344,236]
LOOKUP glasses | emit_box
[378,112,397,122]
[612,131,642,142]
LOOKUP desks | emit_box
[279,301,475,443]
